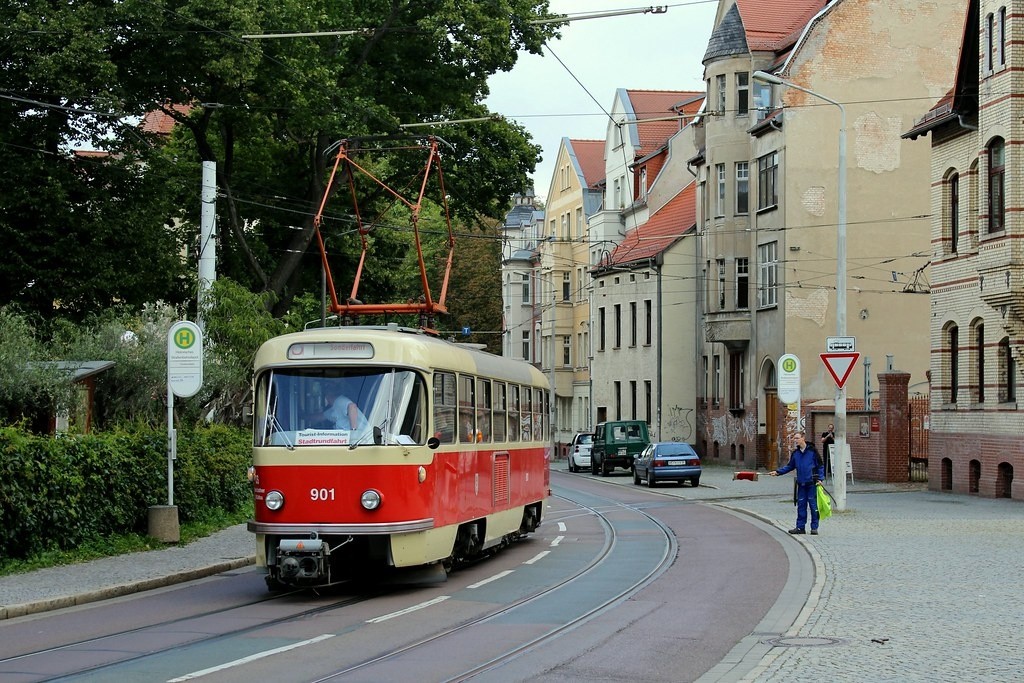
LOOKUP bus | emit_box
[246,328,555,598]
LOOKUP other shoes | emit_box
[811,528,818,534]
[789,528,805,534]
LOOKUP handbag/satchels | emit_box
[816,482,832,521]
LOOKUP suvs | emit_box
[589,420,650,478]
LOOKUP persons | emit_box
[619,425,640,441]
[769,430,825,535]
[436,402,544,446]
[821,424,835,478]
[302,380,369,450]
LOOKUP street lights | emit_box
[751,69,851,514]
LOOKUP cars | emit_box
[632,442,702,487]
[566,433,595,472]
